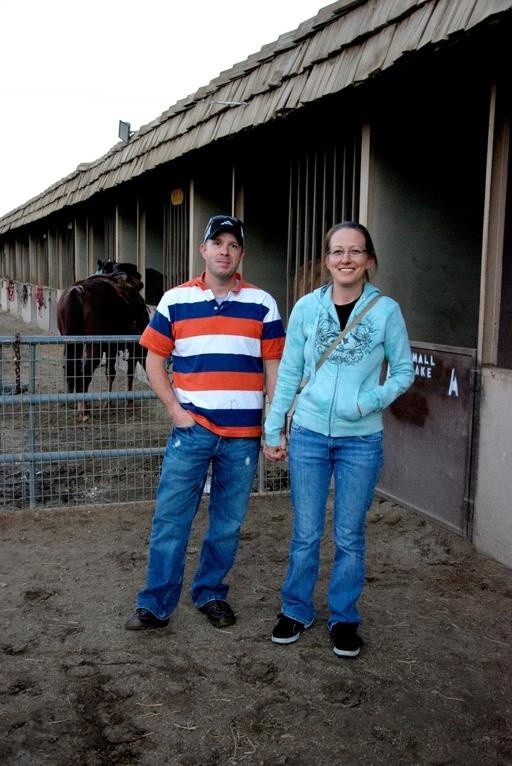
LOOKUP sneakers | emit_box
[271,613,315,643]
[327,620,364,659]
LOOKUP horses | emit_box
[55,272,153,422]
[93,257,166,306]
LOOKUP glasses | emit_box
[325,248,367,258]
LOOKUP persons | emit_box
[124,215,288,631]
[263,221,415,658]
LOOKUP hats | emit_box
[201,214,246,248]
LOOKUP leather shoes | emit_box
[199,600,236,627]
[124,608,170,630]
